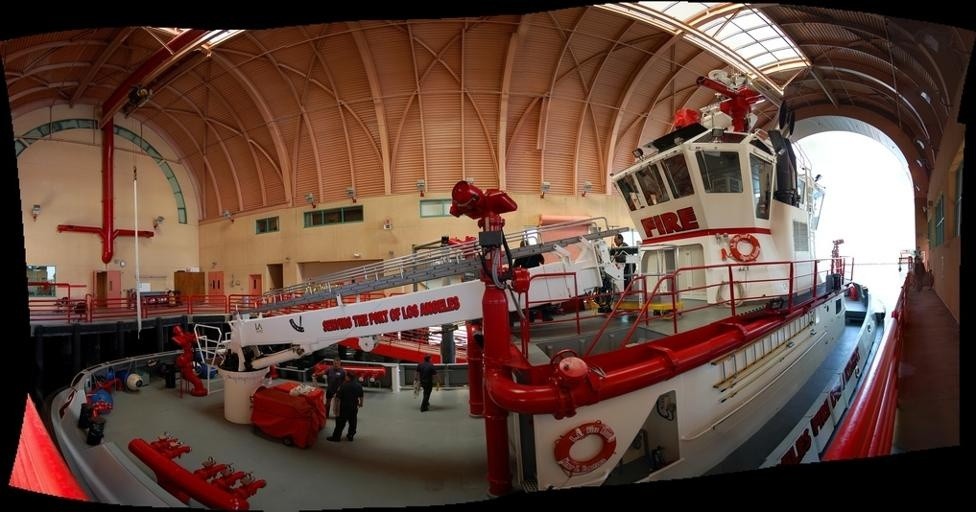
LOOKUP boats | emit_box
[41,59,911,509]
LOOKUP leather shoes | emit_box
[326,436,341,442]
[346,434,354,441]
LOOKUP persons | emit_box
[414,355,441,412]
[316,356,364,441]
[512,240,554,321]
[614,233,639,290]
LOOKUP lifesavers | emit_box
[729,234,760,262]
[847,283,859,300]
[555,422,617,473]
